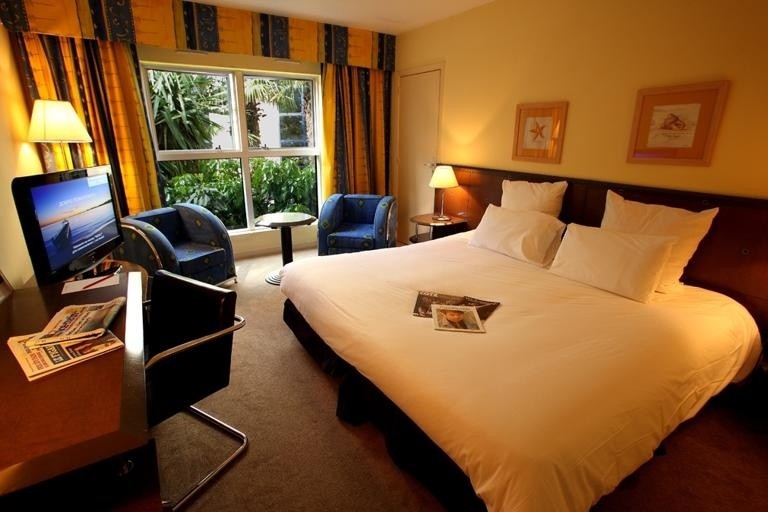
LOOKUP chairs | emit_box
[119,203,238,286]
[145,269,248,511]
[318,193,397,256]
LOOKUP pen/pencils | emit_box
[83,273,114,289]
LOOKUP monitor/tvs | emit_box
[11,164,125,289]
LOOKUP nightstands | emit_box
[407,213,468,244]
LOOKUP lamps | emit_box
[26,99,95,170]
[429,165,458,220]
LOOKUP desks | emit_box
[0,271,160,511]
[254,212,317,285]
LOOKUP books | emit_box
[455,296,500,324]
[431,306,489,337]
[414,291,463,318]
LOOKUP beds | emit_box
[280,163,768,512]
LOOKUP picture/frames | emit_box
[626,80,731,167]
[512,102,568,163]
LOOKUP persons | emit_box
[439,310,478,329]
[74,333,115,353]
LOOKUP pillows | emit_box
[547,223,676,303]
[501,180,569,218]
[601,190,719,294]
[468,203,566,270]
